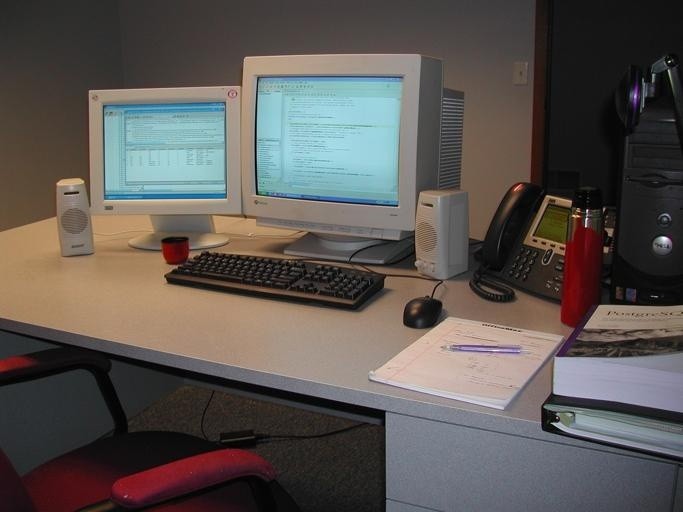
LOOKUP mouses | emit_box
[404,297,442,328]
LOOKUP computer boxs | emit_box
[610,103,683,306]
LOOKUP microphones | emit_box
[618,58,683,136]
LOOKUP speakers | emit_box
[56,178,94,256]
[414,187,469,280]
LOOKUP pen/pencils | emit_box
[440,344,532,354]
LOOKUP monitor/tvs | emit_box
[89,86,241,251]
[242,53,464,264]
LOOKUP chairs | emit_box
[0,347,299,512]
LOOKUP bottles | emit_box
[560,189,603,327]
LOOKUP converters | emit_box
[220,431,257,449]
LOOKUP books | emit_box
[368,315,566,412]
[541,302,683,413]
[535,395,683,460]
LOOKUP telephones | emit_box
[469,182,619,303]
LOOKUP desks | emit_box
[0,214,683,512]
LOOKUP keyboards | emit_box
[164,251,386,310]
